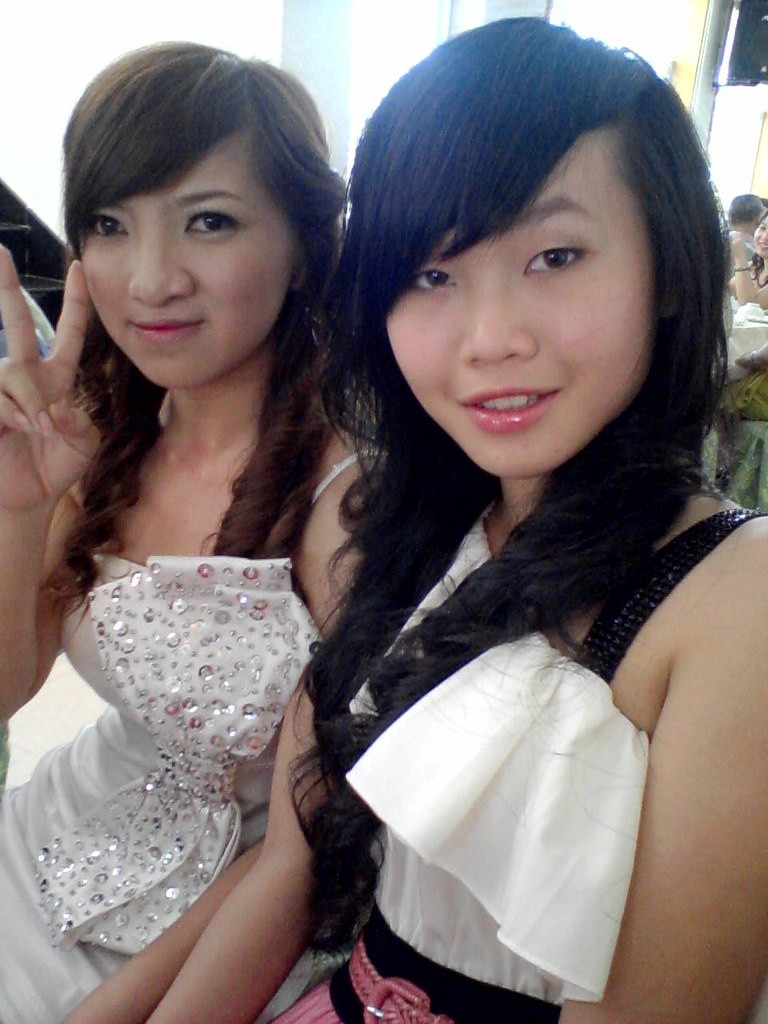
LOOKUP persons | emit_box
[0,18,768,1024]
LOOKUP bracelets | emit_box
[735,267,750,272]
